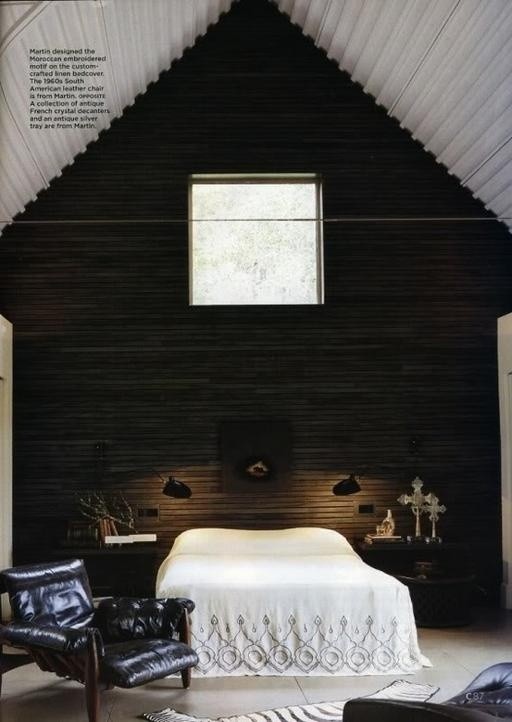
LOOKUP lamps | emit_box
[332,467,372,496]
[146,464,193,499]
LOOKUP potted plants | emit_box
[70,485,140,551]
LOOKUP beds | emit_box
[155,525,421,675]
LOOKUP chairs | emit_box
[0,556,199,722]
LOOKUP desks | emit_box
[356,540,437,577]
[88,552,154,597]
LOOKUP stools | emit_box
[392,575,473,628]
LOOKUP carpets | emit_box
[135,679,441,721]
